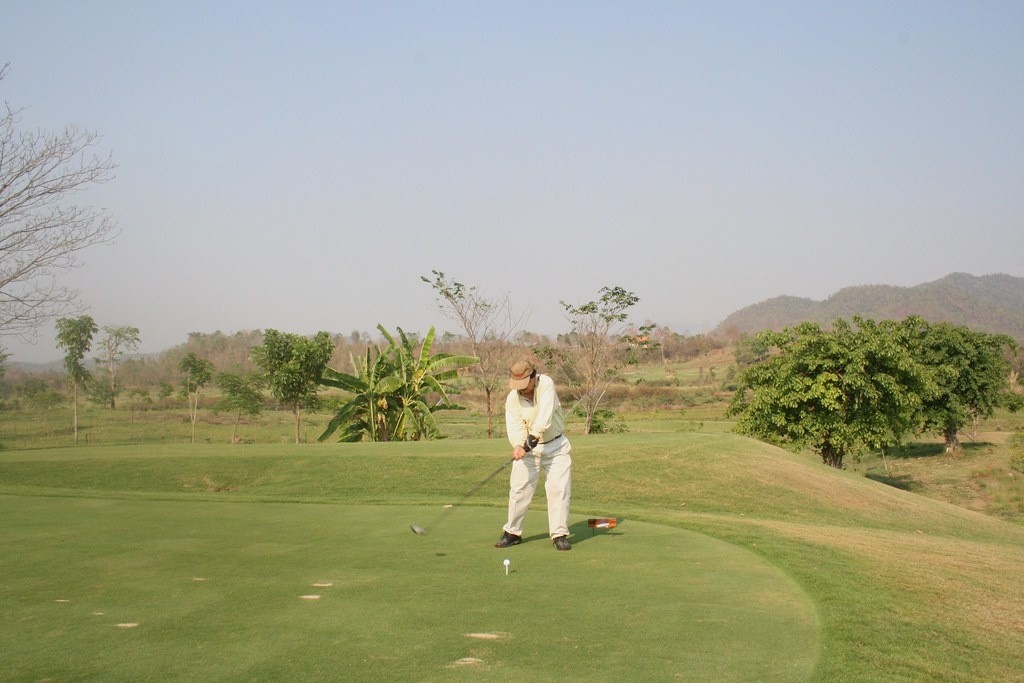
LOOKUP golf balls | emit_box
[503,559,511,566]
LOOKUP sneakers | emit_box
[553,535,570,550]
[495,531,522,548]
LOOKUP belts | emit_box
[539,433,562,445]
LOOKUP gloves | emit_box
[524,435,538,452]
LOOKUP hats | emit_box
[508,360,533,389]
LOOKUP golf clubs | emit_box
[410,435,540,536]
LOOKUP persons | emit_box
[494,360,572,550]
